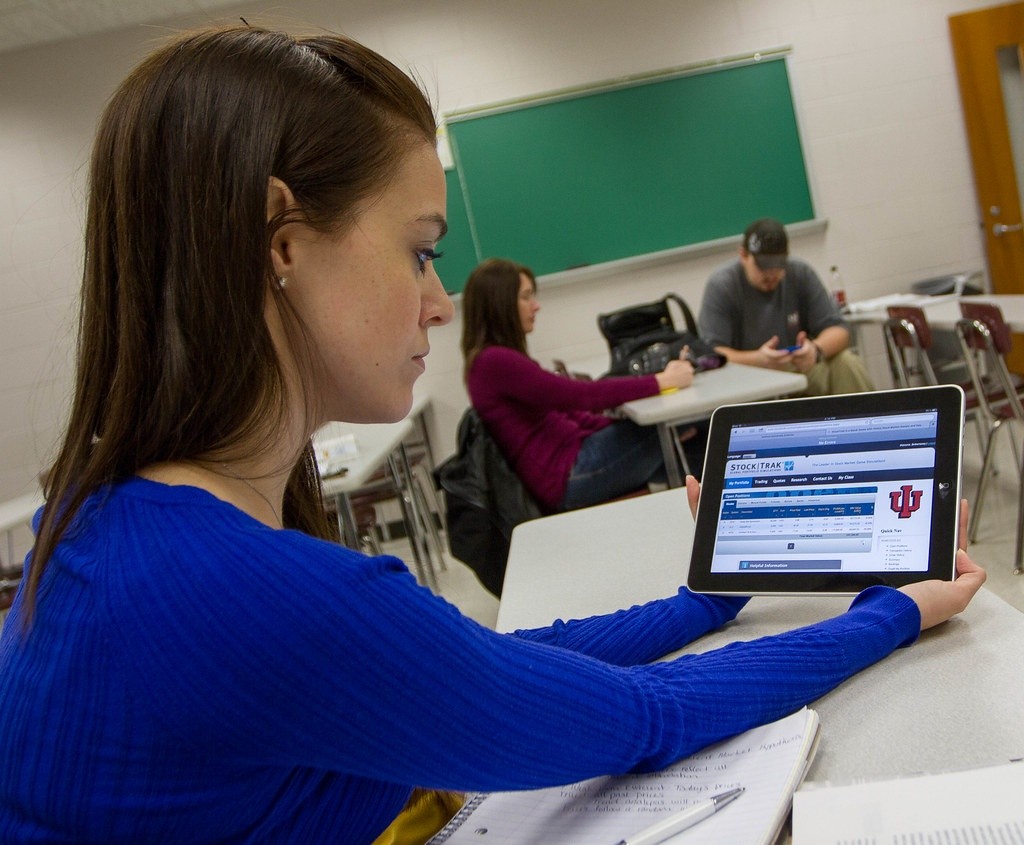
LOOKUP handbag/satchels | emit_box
[621,334,727,377]
[596,295,697,375]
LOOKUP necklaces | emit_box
[205,449,285,530]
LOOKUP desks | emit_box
[493,485,1024,845]
[0,492,46,566]
[572,355,809,489]
[842,294,1024,334]
[309,395,445,593]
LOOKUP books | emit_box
[421,698,1024,843]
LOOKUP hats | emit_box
[744,219,788,267]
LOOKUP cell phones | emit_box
[787,346,802,352]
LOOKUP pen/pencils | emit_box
[679,345,688,361]
[615,787,746,845]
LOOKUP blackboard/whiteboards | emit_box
[426,44,830,305]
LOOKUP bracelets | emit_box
[812,341,823,365]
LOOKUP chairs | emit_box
[364,451,448,542]
[954,301,1024,574]
[325,472,447,572]
[881,302,1024,476]
[430,406,653,600]
[327,505,383,556]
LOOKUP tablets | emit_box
[687,383,967,595]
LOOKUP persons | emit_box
[0,16,986,844]
[696,217,877,397]
[461,256,693,515]
